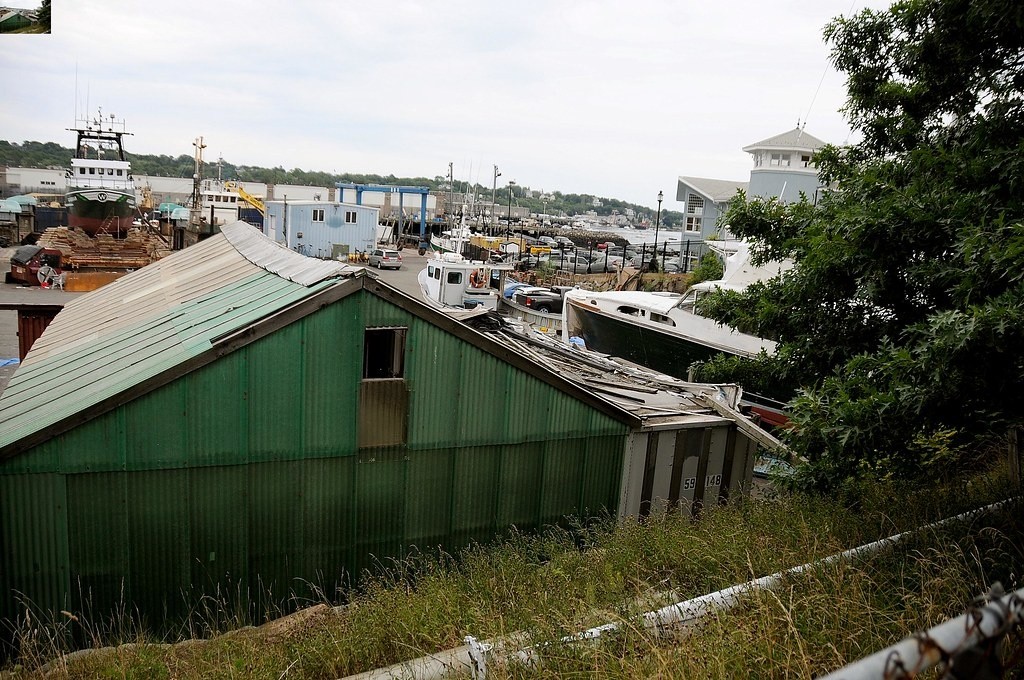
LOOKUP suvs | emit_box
[367,248,403,270]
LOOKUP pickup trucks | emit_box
[516,285,577,314]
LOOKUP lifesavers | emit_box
[471,271,487,288]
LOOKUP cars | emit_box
[503,283,535,300]
[515,233,681,276]
[518,215,589,232]
[589,254,630,273]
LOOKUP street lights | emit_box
[542,201,548,228]
[506,181,517,243]
[650,191,664,261]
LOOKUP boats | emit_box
[416,161,514,321]
[158,135,264,226]
[561,284,816,482]
[55,61,145,238]
